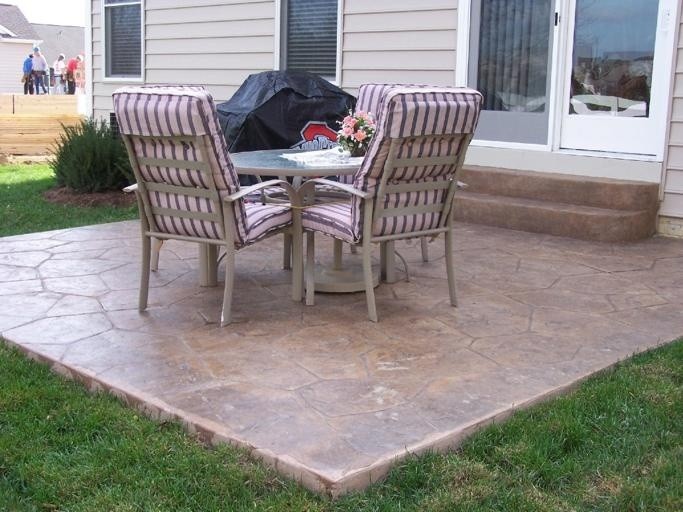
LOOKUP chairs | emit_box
[124,81,298,272]
[301,89,485,324]
[304,81,458,282]
[109,85,304,328]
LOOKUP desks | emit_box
[220,147,391,294]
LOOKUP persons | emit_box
[23,54,34,94]
[52,55,66,93]
[30,46,48,95]
[66,56,82,94]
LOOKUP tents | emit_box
[215,65,359,183]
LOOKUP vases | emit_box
[348,145,366,156]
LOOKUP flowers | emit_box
[332,103,376,157]
